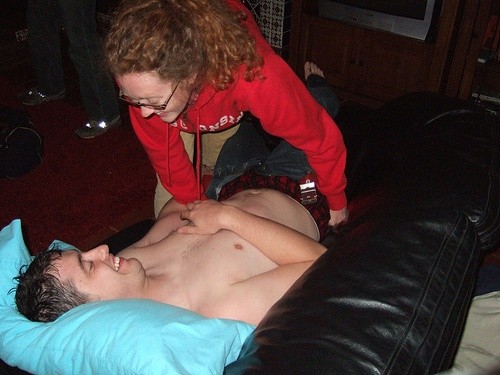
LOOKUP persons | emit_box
[105,0,348,227]
[15,61,333,327]
[18,0,121,137]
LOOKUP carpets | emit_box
[0,58,157,254]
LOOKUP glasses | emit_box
[117,79,182,111]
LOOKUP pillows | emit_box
[0,219,254,375]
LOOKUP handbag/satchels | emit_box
[0,105,46,180]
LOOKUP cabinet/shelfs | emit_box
[288,0,500,110]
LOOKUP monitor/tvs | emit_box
[319,0,441,43]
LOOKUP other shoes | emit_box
[16,81,67,105]
[74,114,121,139]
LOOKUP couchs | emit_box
[0,89,500,375]
